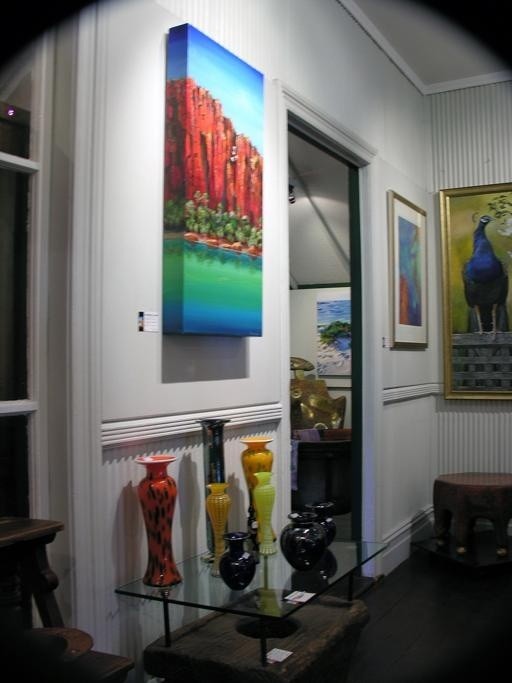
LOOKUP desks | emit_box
[299,440,350,502]
[114,541,389,683]
[0,516,135,683]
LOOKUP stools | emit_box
[434,472,512,557]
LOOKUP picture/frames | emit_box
[387,189,429,348]
[439,183,512,401]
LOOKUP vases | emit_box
[136,419,337,590]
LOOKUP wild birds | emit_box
[460,211,509,386]
